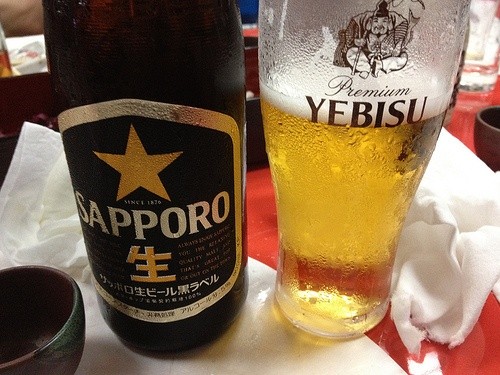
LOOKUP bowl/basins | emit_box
[475,104,500,172]
[0,264,84,374]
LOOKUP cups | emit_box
[459,1,499,93]
[257,0,471,339]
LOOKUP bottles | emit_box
[41,0,249,354]
